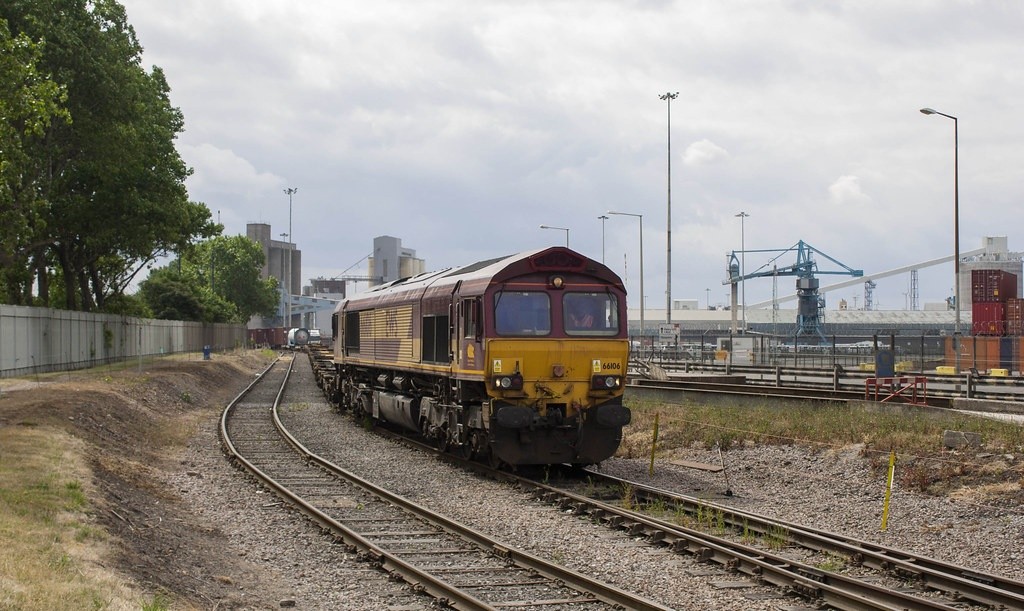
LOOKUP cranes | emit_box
[721,239,864,346]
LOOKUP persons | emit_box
[566,298,594,328]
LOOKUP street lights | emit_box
[920,108,962,374]
[598,216,610,264]
[283,187,297,328]
[736,212,751,335]
[607,210,646,360]
[704,288,712,309]
[657,89,681,360]
[279,232,290,327]
[539,223,569,249]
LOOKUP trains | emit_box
[250,325,310,350]
[303,244,631,473]
